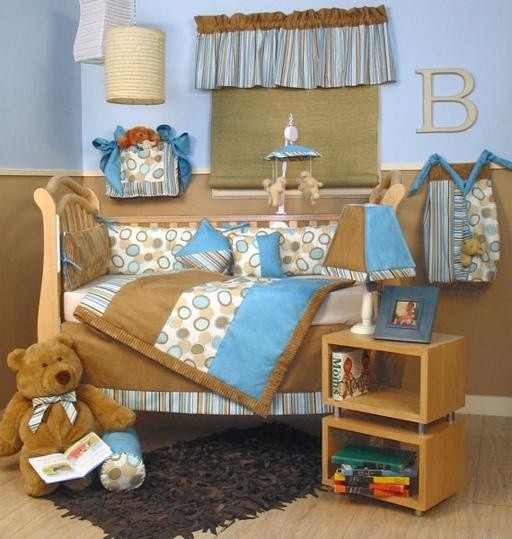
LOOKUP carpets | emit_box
[37,422,328,539]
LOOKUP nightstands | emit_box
[322,330,468,516]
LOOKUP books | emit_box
[330,444,419,499]
[331,347,373,401]
[30,431,114,484]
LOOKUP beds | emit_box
[33,176,404,418]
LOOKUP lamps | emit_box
[104,26,165,104]
[74,0,137,64]
[322,204,416,336]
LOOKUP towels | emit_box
[421,180,500,284]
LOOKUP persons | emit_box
[394,302,415,325]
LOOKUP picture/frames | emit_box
[374,285,440,345]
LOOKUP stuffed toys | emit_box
[139,139,156,150]
[118,125,160,147]
[459,232,484,267]
[1,334,146,498]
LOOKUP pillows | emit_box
[229,233,284,278]
[172,219,232,275]
